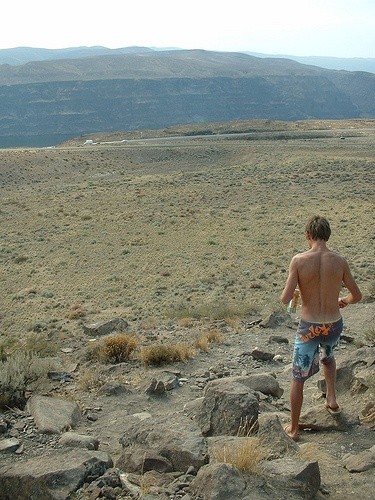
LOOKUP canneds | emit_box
[287,289,299,313]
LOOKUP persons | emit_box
[281,216,363,442]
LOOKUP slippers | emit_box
[324,402,341,415]
[284,426,299,441]
